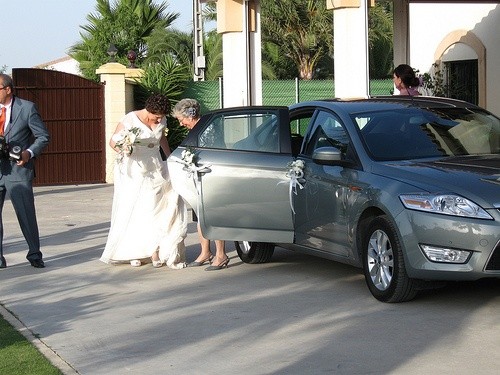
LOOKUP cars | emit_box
[164,95,500,303]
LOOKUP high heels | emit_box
[204,256,230,271]
[187,254,214,267]
[130,260,141,267]
[150,250,163,267]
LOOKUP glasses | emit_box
[179,116,185,124]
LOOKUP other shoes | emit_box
[31,259,44,268]
[0,256,7,268]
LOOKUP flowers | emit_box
[163,127,170,137]
[112,126,141,157]
[180,147,201,170]
[289,159,306,176]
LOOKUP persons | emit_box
[0,73,50,268]
[100,94,187,269]
[171,99,229,271]
[393,64,420,96]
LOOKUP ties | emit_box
[0,107,6,136]
